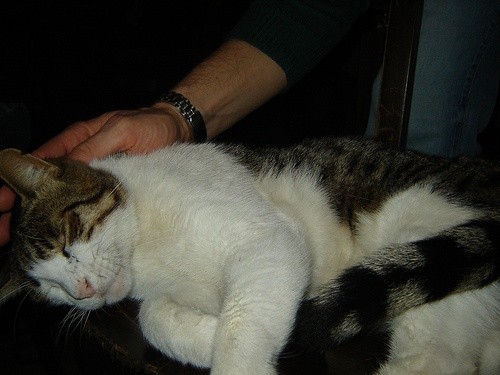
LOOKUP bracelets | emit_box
[159,91,209,146]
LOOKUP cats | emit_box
[0,137,500,375]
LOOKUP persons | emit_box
[1,1,500,250]
